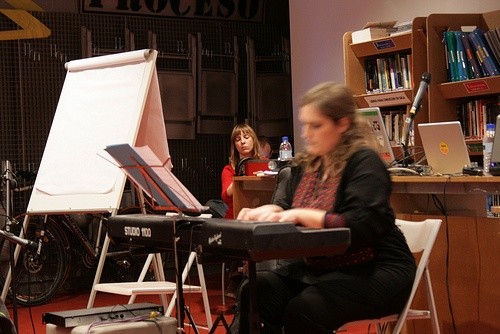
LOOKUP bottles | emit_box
[482,124,496,175]
[279,136,292,160]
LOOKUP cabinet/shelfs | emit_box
[426,8,500,161]
[341,13,426,164]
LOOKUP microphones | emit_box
[409,72,431,115]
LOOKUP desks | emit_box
[233,173,500,333]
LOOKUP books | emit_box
[364,53,413,94]
[456,99,500,139]
[443,25,500,82]
[381,111,415,148]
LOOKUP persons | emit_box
[238,80,417,334]
[221,124,273,220]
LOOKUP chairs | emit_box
[86,211,214,331]
[344,217,442,334]
[81,24,291,143]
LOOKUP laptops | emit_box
[418,121,472,175]
[356,106,423,168]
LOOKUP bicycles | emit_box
[0,159,171,307]
